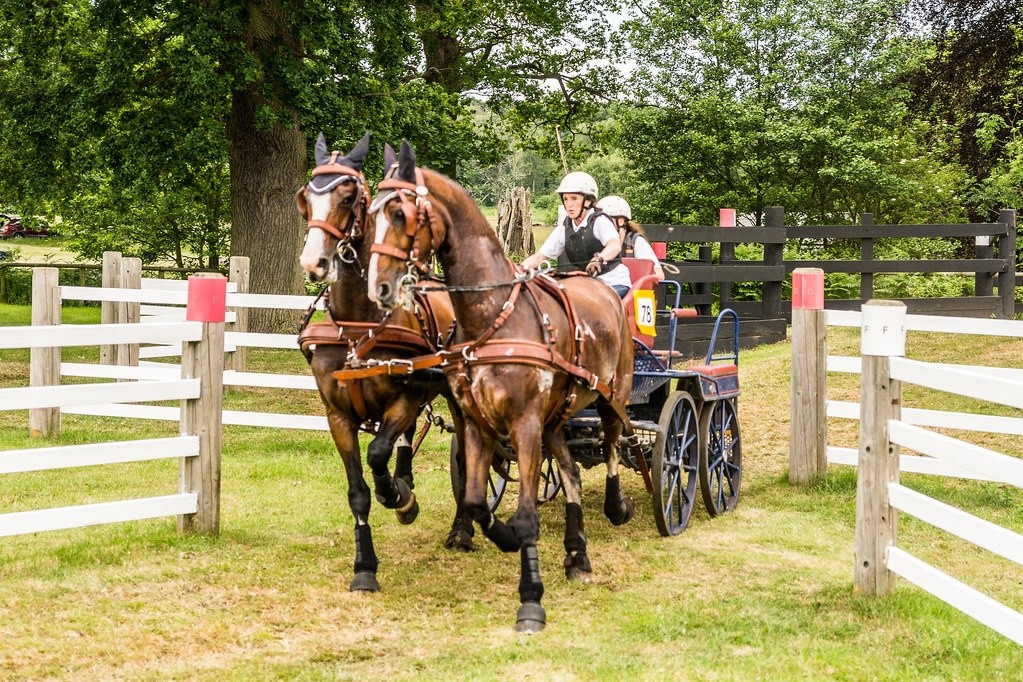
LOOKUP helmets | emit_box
[555,172,599,201]
[595,195,631,220]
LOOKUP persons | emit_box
[516,171,629,301]
[595,196,667,280]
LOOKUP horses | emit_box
[366,138,635,633]
[296,132,476,593]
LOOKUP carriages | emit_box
[297,132,744,637]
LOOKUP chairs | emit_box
[617,255,657,350]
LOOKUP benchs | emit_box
[684,361,740,379]
[634,348,685,361]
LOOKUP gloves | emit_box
[585,252,605,276]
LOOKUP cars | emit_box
[0,215,65,242]
